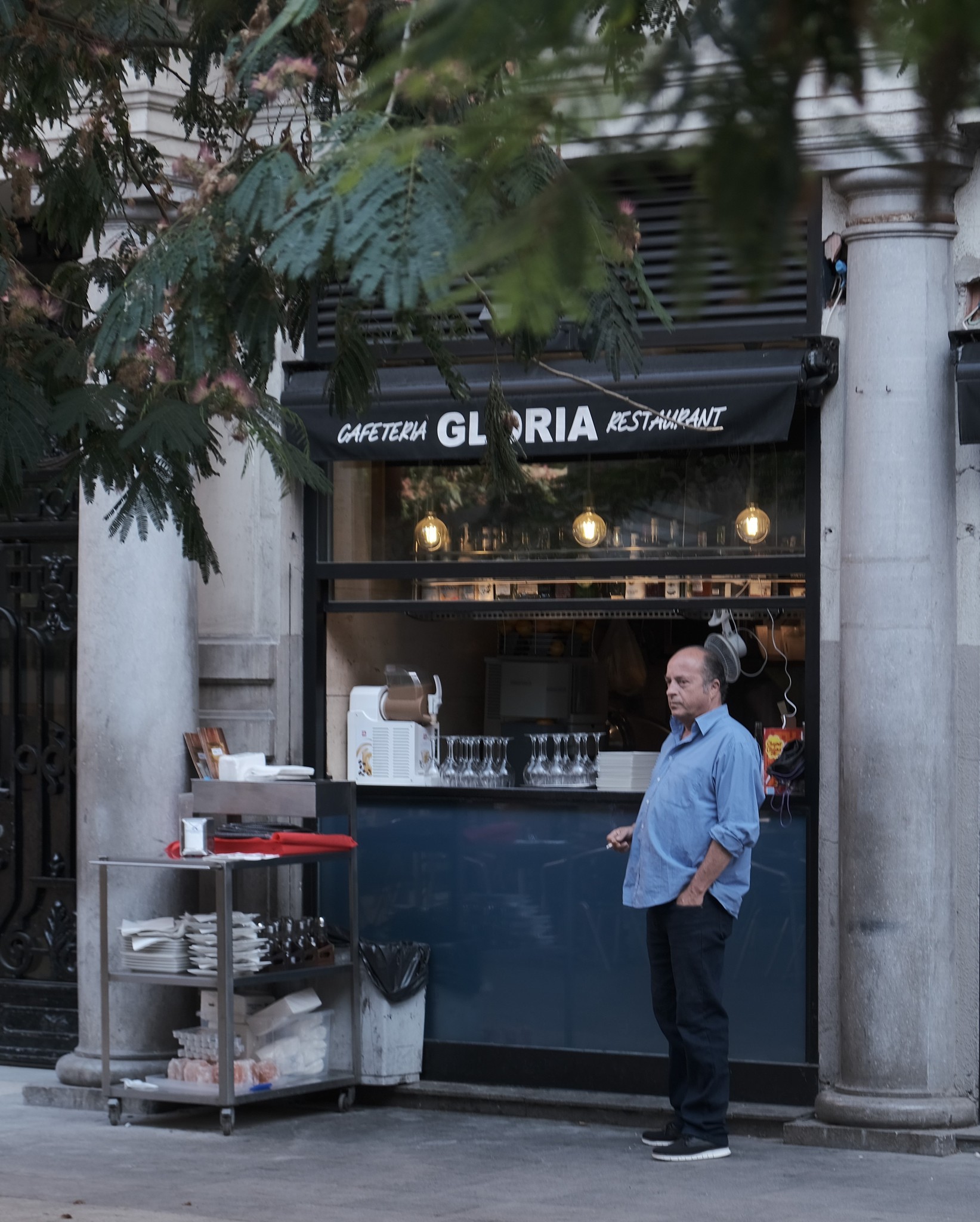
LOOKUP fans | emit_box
[704,608,747,683]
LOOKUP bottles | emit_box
[421,518,805,601]
[255,916,329,965]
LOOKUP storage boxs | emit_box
[200,989,274,1025]
[208,1022,264,1059]
[246,988,322,1037]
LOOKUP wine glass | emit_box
[424,735,515,787]
[523,732,607,785]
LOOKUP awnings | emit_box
[282,336,831,463]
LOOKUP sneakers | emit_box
[641,1121,683,1147]
[651,1134,731,1161]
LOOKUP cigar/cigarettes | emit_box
[606,842,613,849]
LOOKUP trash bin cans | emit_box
[329,934,430,1086]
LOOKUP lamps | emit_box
[414,497,448,551]
[571,460,607,548]
[735,447,770,544]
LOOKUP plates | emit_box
[178,913,272,976]
[115,926,196,973]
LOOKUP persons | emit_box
[607,647,767,1161]
